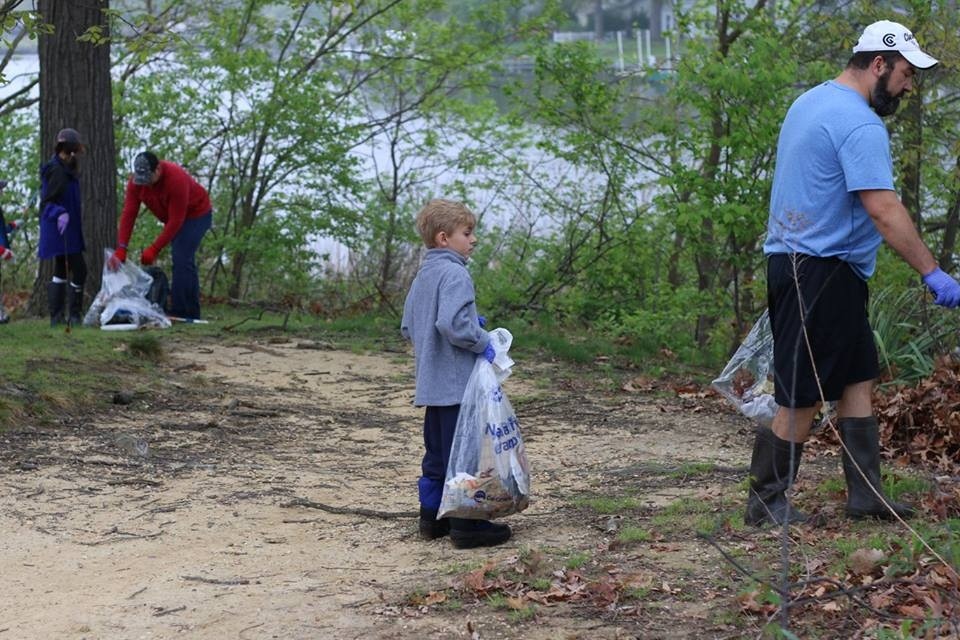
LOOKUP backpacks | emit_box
[140,266,168,315]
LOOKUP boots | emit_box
[836,417,914,520]
[745,426,803,527]
[418,478,450,541]
[449,520,510,549]
[67,281,83,325]
[48,281,67,327]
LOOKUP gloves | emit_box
[923,266,960,309]
[107,247,126,274]
[478,317,488,327]
[0,247,14,261]
[9,219,25,229]
[482,342,495,363]
[57,213,70,235]
[141,246,159,266]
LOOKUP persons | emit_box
[744,21,960,526]
[40,128,87,328]
[108,152,213,320]
[0,181,24,325]
[400,197,512,549]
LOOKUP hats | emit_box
[853,21,939,69]
[133,152,159,184]
[58,128,82,144]
